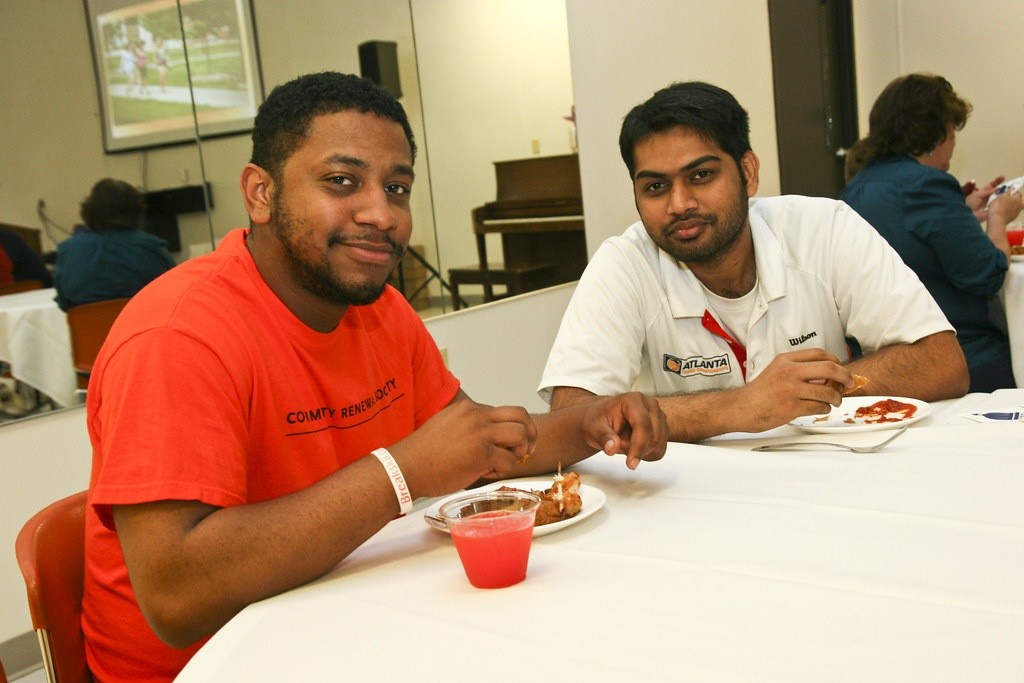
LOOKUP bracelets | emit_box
[371,446,416,517]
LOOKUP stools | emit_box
[447,263,552,311]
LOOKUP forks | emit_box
[751,426,907,454]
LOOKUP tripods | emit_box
[391,248,469,311]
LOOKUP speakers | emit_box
[357,41,403,101]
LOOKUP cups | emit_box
[440,490,541,591]
[1003,221,1024,248]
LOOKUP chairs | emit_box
[62,298,137,408]
[14,489,98,683]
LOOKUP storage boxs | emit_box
[389,245,428,310]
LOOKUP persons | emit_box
[80,72,669,681]
[840,72,1024,394]
[537,79,971,433]
[47,178,181,313]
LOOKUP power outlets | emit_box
[23,191,52,211]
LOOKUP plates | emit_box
[422,476,606,542]
[786,394,933,435]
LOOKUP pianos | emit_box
[470,153,588,302]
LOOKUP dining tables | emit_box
[164,384,1024,681]
